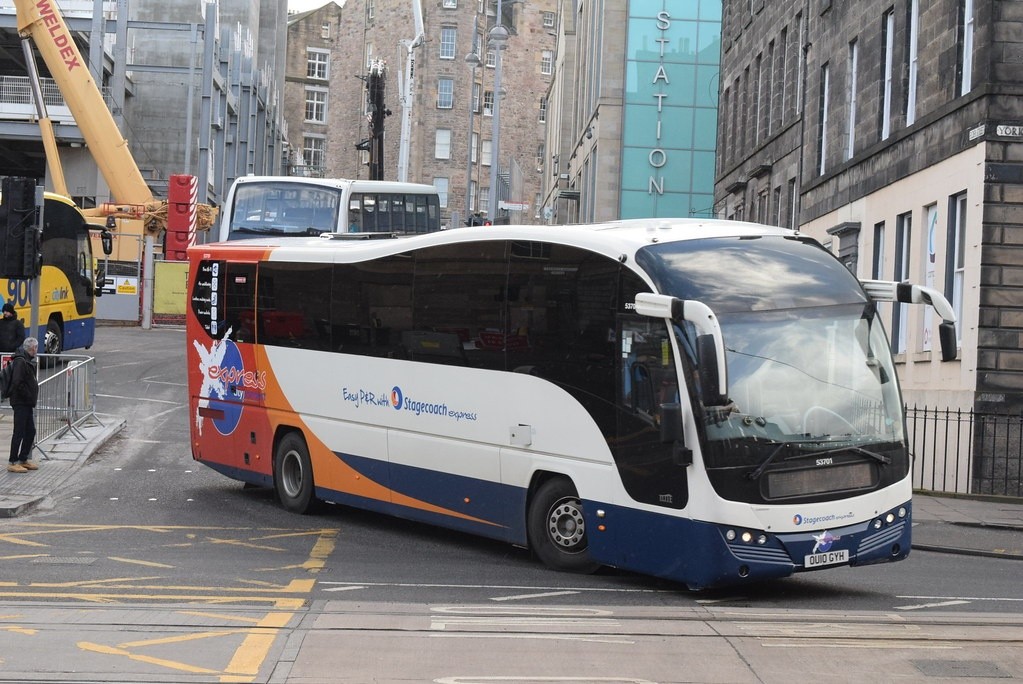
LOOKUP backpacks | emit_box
[0,357,28,398]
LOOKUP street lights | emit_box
[463,0,484,227]
[486,0,512,226]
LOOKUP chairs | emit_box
[285,208,435,233]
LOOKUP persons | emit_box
[0,303,26,353]
[6,337,39,473]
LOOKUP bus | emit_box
[0,182,113,367]
[187,216,958,588]
[219,174,442,241]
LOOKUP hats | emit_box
[2,303,14,314]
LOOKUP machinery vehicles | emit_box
[355,0,427,182]
[6,0,218,262]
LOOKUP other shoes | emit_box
[8,464,27,473]
[20,462,38,469]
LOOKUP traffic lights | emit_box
[463,217,472,227]
[473,218,483,227]
[483,219,492,226]
